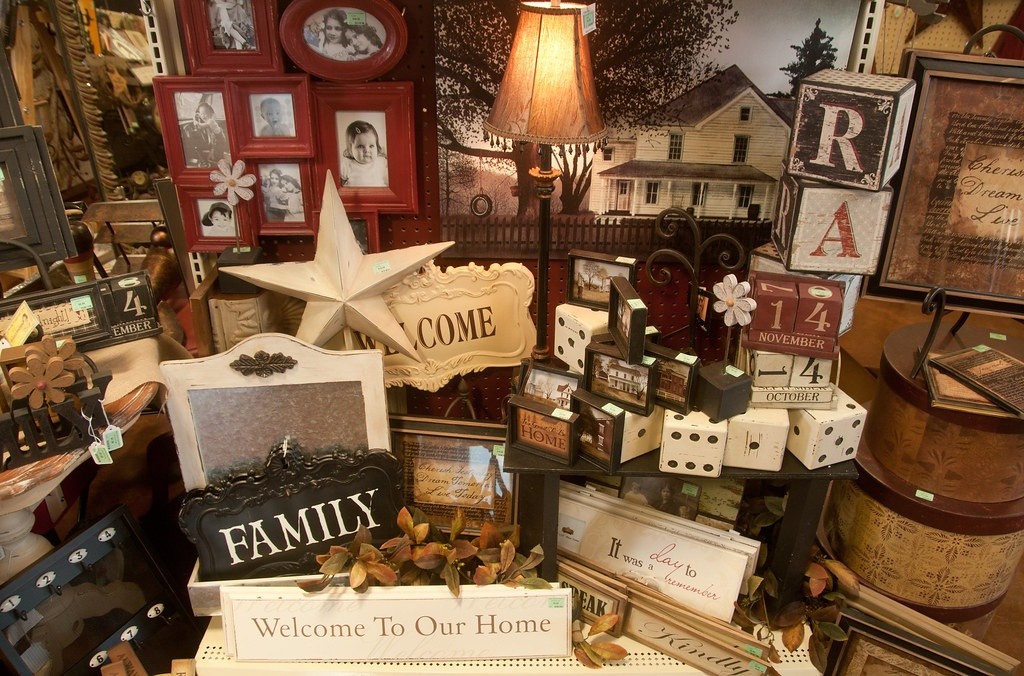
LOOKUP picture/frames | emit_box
[564,248,640,312]
[928,344,1024,419]
[516,358,584,414]
[388,414,520,538]
[644,341,701,416]
[278,0,409,84]
[860,48,1024,320]
[618,476,703,521]
[584,341,658,417]
[916,345,1020,419]
[311,207,381,256]
[311,82,419,215]
[225,72,318,159]
[152,75,246,185]
[175,185,259,253]
[177,0,286,78]
[0,0,129,272]
[242,158,319,236]
[608,276,648,365]
[509,396,582,467]
[686,282,716,335]
[821,579,1022,676]
[569,388,625,475]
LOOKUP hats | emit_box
[202,203,232,226]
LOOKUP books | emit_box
[917,343,1024,421]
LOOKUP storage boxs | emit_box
[814,481,1009,643]
[863,319,1024,502]
[187,556,402,617]
[823,401,1024,608]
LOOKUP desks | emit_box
[0,335,160,676]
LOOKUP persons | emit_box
[623,482,649,508]
[321,8,349,61]
[194,102,226,160]
[651,483,682,517]
[339,120,388,188]
[201,201,235,236]
[257,97,290,137]
[261,169,287,222]
[207,0,257,50]
[278,174,304,222]
[343,21,383,61]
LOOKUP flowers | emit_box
[210,159,257,206]
[9,335,85,410]
[711,273,757,326]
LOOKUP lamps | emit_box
[482,0,609,362]
